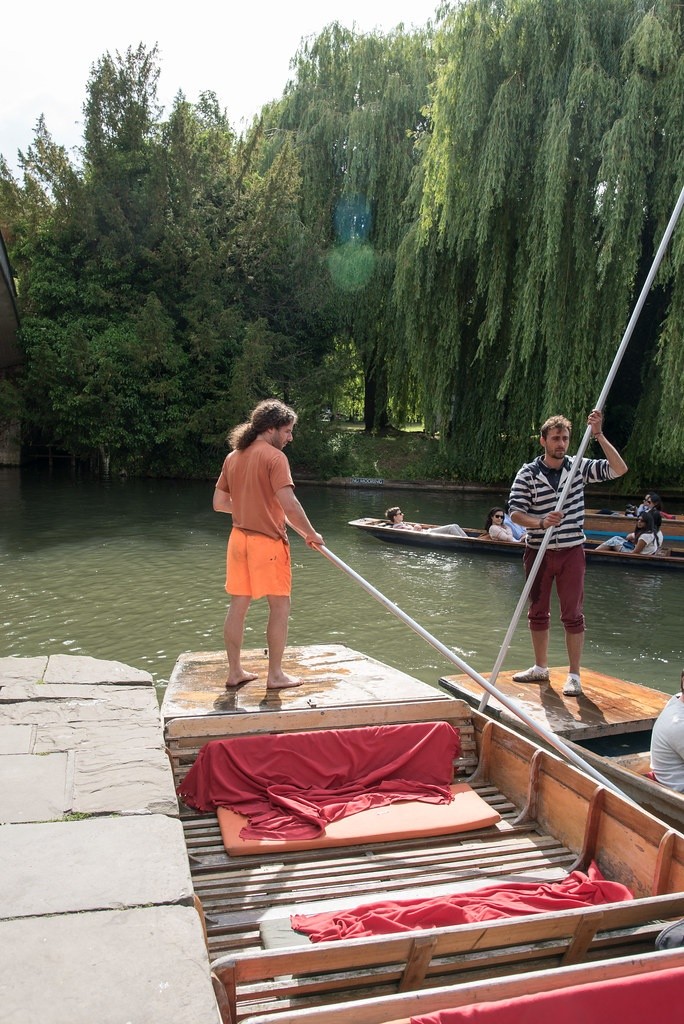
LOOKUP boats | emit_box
[438,660,684,833]
[159,641,683,1023]
[348,516,684,573]
[582,508,684,547]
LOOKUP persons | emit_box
[595,492,679,557]
[508,408,628,698]
[213,398,325,690]
[385,507,469,538]
[483,501,527,544]
[650,669,684,794]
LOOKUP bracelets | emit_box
[539,516,546,531]
[594,431,603,435]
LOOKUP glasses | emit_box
[494,515,504,519]
[644,499,648,502]
[647,501,651,504]
[636,518,644,523]
[396,512,402,515]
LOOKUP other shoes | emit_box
[563,678,583,695]
[512,667,549,682]
